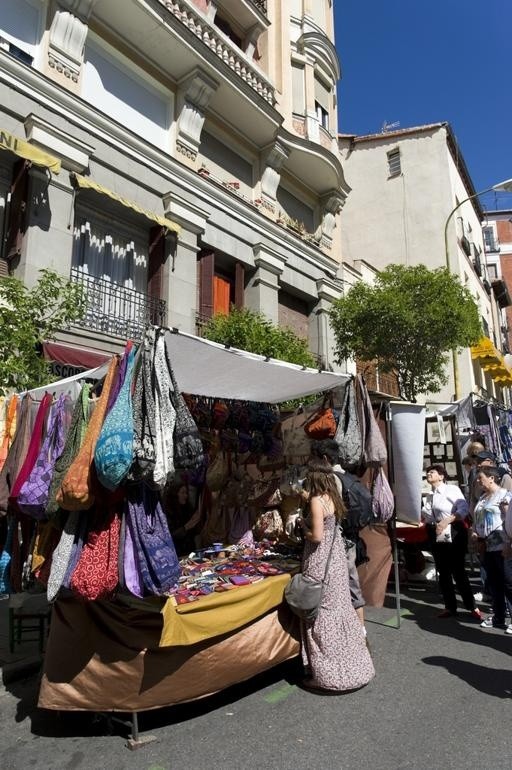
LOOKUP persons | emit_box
[164,482,209,556]
[296,438,368,643]
[297,459,376,693]
[418,441,512,636]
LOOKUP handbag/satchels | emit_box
[206,398,283,544]
[371,467,394,524]
[336,379,362,469]
[281,458,307,497]
[362,380,388,465]
[302,390,336,441]
[436,523,452,542]
[284,573,325,626]
[283,406,311,457]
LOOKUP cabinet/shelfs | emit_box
[54,556,303,742]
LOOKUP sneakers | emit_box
[480,617,506,628]
[472,610,485,621]
[506,624,512,633]
[436,608,457,618]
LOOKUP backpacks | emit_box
[338,470,374,530]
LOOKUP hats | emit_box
[472,451,496,462]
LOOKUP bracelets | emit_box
[299,521,308,532]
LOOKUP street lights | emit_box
[443,176,512,403]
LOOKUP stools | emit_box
[9,603,52,654]
